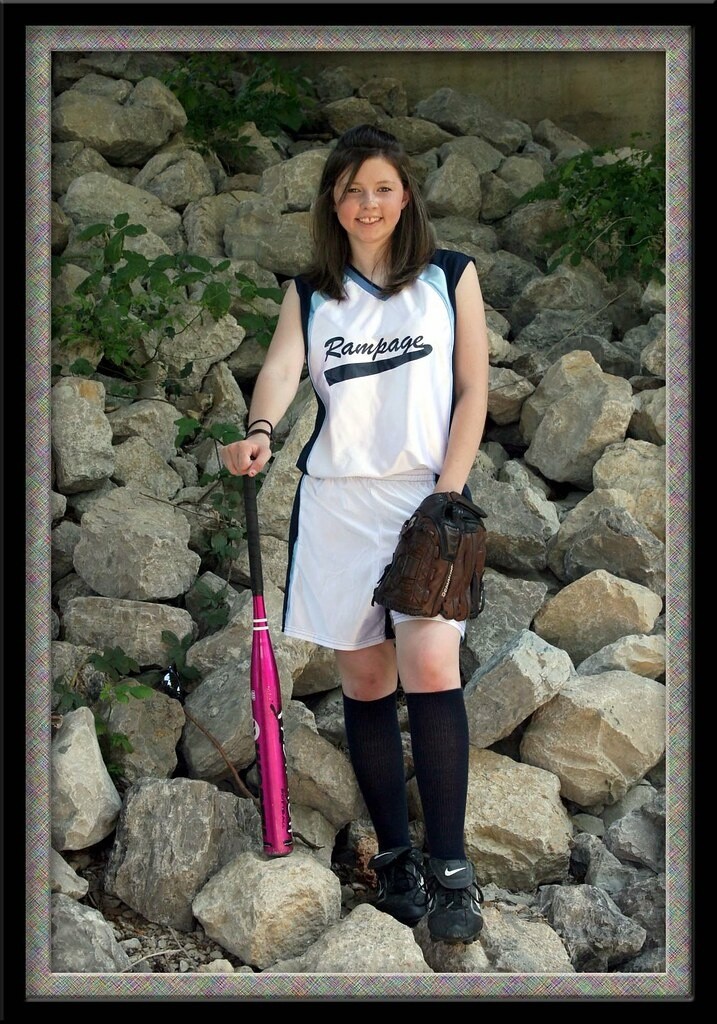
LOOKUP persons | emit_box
[221,125,488,943]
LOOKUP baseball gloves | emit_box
[370,486,491,623]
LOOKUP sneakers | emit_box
[367,846,427,924]
[421,853,484,944]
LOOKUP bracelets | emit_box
[244,419,273,439]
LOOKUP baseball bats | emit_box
[238,469,296,861]
